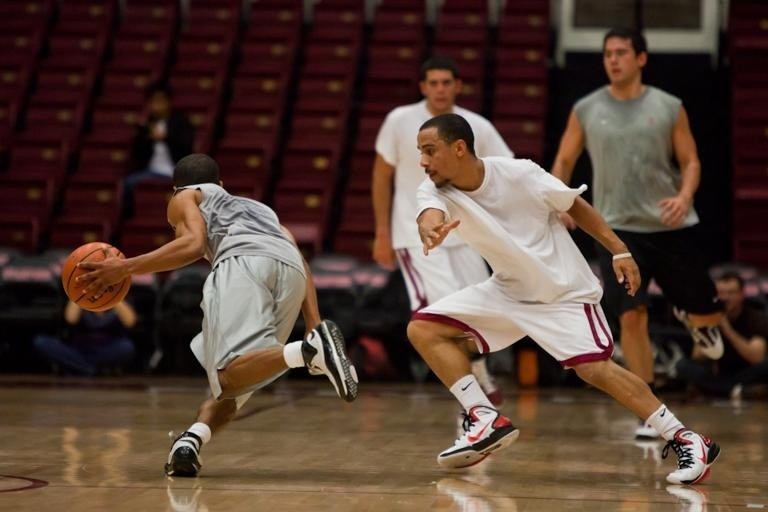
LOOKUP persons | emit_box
[371,56,515,410]
[406,114,721,484]
[74,152,359,478]
[120,81,193,220]
[549,27,725,440]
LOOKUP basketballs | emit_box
[63,242,132,312]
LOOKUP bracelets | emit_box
[612,252,631,261]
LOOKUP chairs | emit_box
[721,1,768,276]
[0,1,558,268]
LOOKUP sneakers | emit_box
[163,430,202,478]
[483,383,505,409]
[302,318,360,402]
[663,428,721,485]
[634,417,661,440]
[435,405,521,469]
[673,304,726,362]
